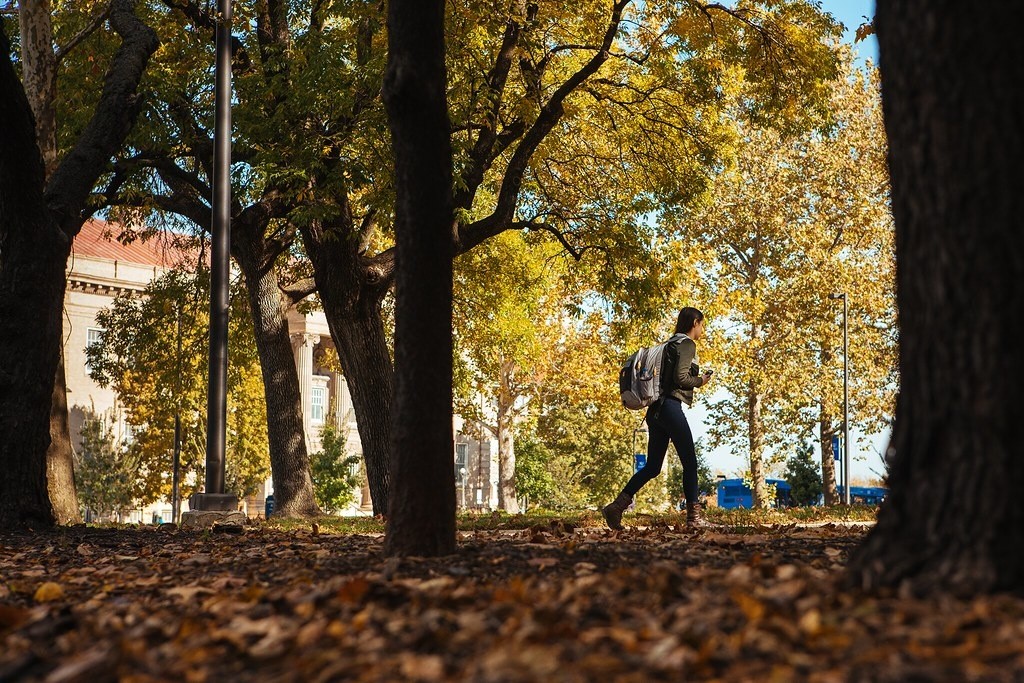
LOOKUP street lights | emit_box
[633,426,648,476]
[828,292,852,506]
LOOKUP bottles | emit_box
[641,368,651,380]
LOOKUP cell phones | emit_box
[705,370,713,376]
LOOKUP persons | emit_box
[697,492,712,511]
[626,498,635,513]
[676,492,686,513]
[601,307,721,530]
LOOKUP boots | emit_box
[686,502,708,530]
[601,492,633,532]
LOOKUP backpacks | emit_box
[619,336,695,410]
